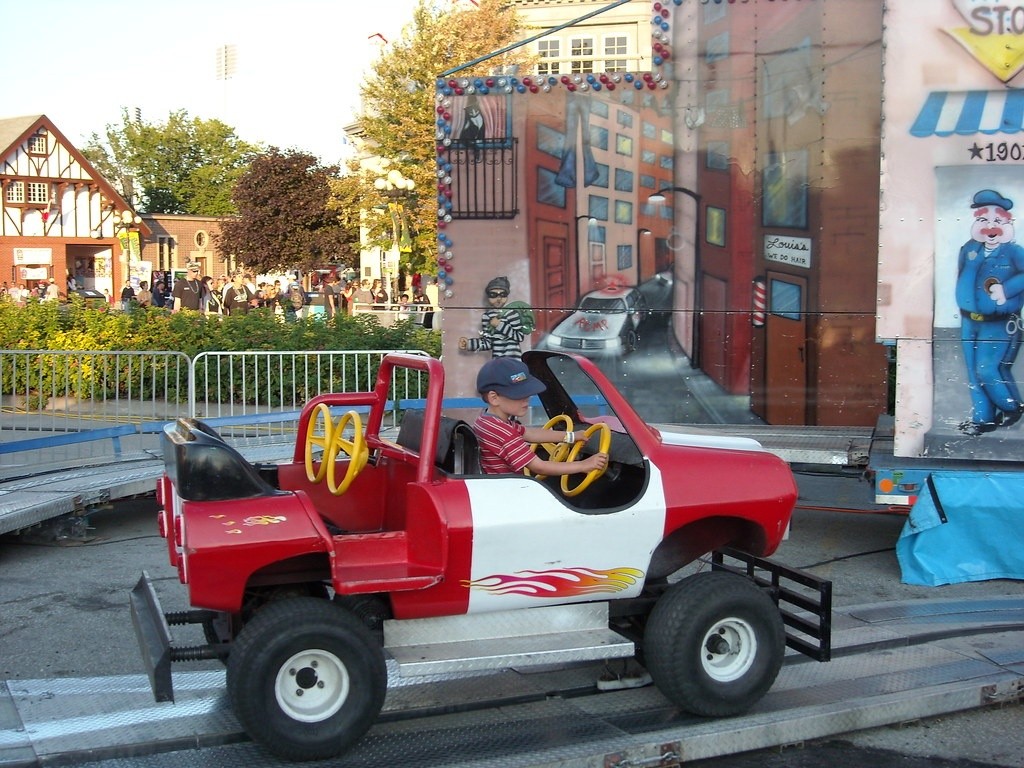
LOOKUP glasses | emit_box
[192,270,198,272]
[207,282,213,285]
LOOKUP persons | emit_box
[0,275,435,331]
[473,357,608,478]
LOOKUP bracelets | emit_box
[565,431,575,444]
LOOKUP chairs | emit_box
[400,407,482,476]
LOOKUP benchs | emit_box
[177,417,340,533]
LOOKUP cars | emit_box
[130,345,833,766]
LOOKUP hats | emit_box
[186,261,198,269]
[476,357,548,400]
[155,280,164,287]
[49,277,54,282]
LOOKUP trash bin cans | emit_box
[74,289,106,310]
